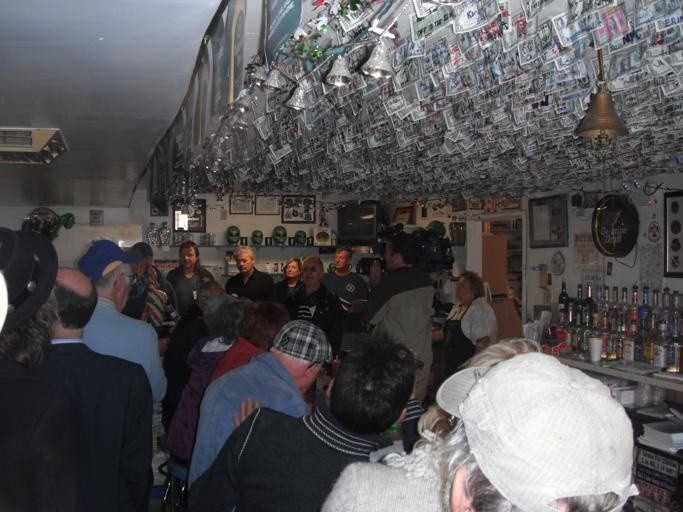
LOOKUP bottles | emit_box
[612,324,623,359]
[592,285,602,320]
[576,283,583,312]
[608,286,620,328]
[570,312,582,352]
[620,287,629,332]
[578,312,592,352]
[638,286,651,329]
[628,285,639,322]
[653,318,669,370]
[623,319,636,361]
[634,318,647,360]
[598,316,609,357]
[667,317,683,373]
[584,284,593,314]
[650,289,660,319]
[642,314,657,365]
[602,286,611,311]
[590,318,599,337]
[658,287,671,329]
[607,319,615,355]
[670,290,683,337]
[560,282,569,324]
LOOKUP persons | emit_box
[168,287,290,505]
[276,255,341,345]
[361,234,435,404]
[165,243,216,321]
[226,246,273,303]
[441,272,498,349]
[324,246,368,314]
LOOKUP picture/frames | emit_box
[528,194,569,248]
[282,196,316,223]
[174,199,206,233]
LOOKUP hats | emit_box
[433,351,641,512]
[74,238,147,282]
[271,319,334,363]
[0,223,60,337]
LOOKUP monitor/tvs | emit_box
[337,199,386,244]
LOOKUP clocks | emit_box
[593,195,640,257]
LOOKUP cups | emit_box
[587,337,603,363]
